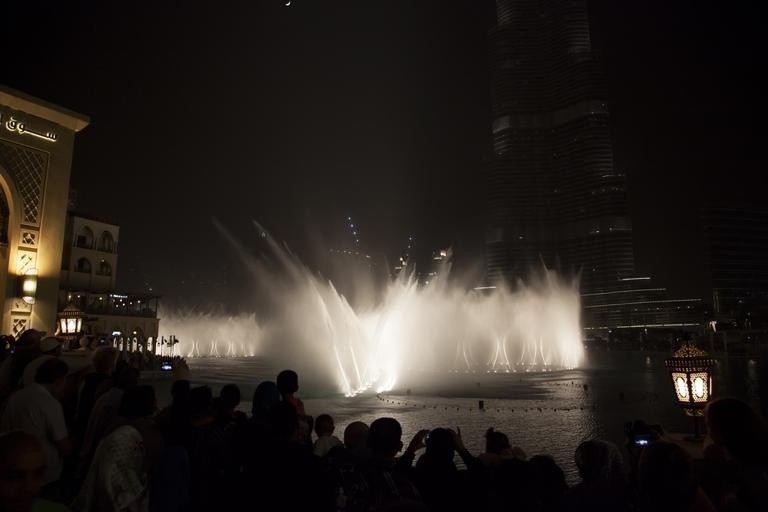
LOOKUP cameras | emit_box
[635,440,648,447]
[160,360,174,371]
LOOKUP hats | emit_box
[41,337,60,353]
